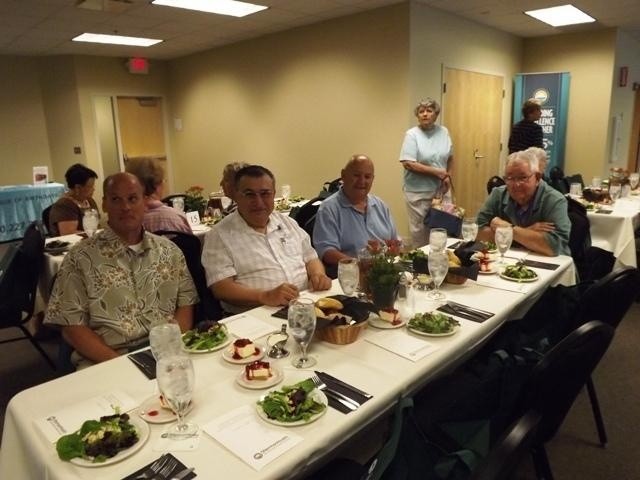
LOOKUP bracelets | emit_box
[509,223,516,230]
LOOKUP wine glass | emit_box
[570,183,582,200]
[609,183,621,201]
[281,184,291,200]
[157,358,198,440]
[149,317,184,362]
[630,172,639,188]
[338,258,359,297]
[461,217,478,242]
[84,208,98,220]
[429,228,447,252]
[82,215,99,238]
[172,197,184,211]
[494,225,513,265]
[288,297,317,370]
[428,250,449,301]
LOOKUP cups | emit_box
[592,176,602,187]
[204,207,221,218]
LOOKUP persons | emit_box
[41,171,201,371]
[398,96,456,247]
[215,160,251,219]
[51,162,97,237]
[506,97,544,159]
[198,164,333,317]
[125,154,192,242]
[523,145,549,183]
[312,153,402,279]
[473,151,572,257]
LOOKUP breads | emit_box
[316,297,345,310]
[445,249,461,265]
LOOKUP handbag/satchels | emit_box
[423,174,465,237]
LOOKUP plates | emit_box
[499,267,538,281]
[222,343,265,364]
[44,241,73,251]
[407,312,457,336]
[237,367,284,389]
[369,305,409,329]
[478,267,497,274]
[274,204,293,212]
[67,415,150,468]
[139,396,197,424]
[181,330,232,353]
[255,386,328,428]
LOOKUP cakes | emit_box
[245,360,271,381]
[477,256,492,271]
[378,307,399,323]
[233,338,261,360]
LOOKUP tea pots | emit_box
[207,192,224,214]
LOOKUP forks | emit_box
[311,375,360,407]
[149,457,177,480]
[129,454,168,480]
[440,304,484,322]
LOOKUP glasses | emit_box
[504,174,534,181]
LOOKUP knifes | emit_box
[314,370,372,399]
[448,300,493,317]
[171,466,196,480]
